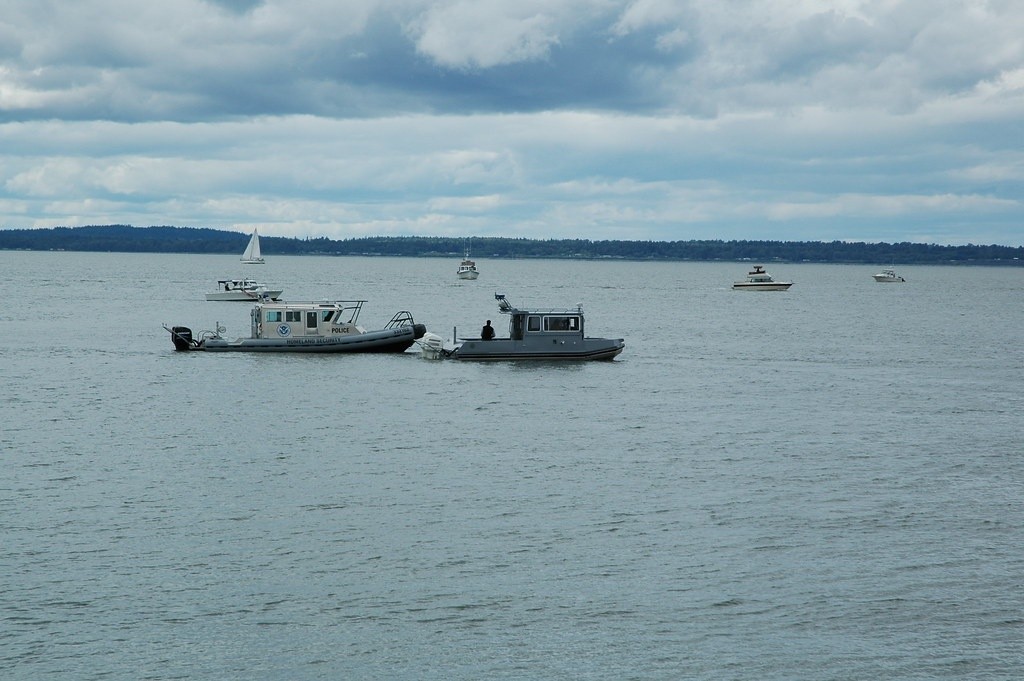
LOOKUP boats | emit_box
[415,294,626,361]
[205,278,283,301]
[732,266,794,291]
[162,294,425,353]
[456,260,480,280]
[872,268,905,283]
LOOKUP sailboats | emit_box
[240,229,265,264]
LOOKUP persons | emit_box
[481,320,495,341]
